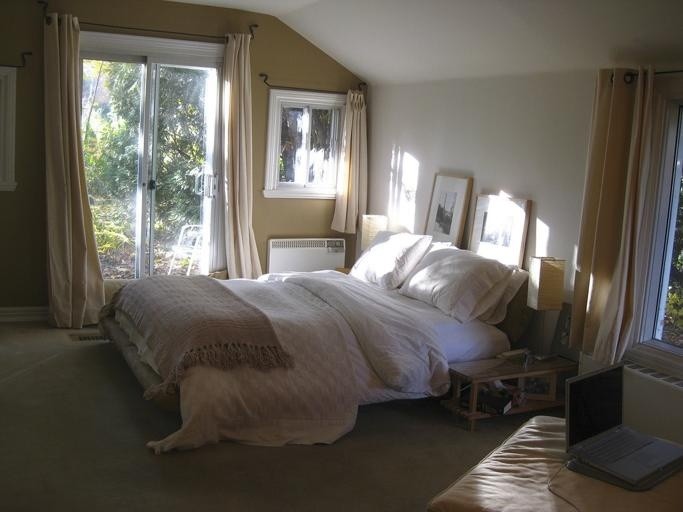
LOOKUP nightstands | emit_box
[441,354,580,436]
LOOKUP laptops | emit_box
[564,362,683,485]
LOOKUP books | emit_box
[459,382,515,416]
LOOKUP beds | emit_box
[102,233,538,444]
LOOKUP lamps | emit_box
[360,214,388,255]
[525,254,566,362]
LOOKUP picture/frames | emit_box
[467,193,531,269]
[424,173,474,251]
[261,88,349,200]
[518,371,558,403]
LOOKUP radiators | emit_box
[265,238,345,273]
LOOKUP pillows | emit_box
[349,233,433,289]
[397,246,510,326]
